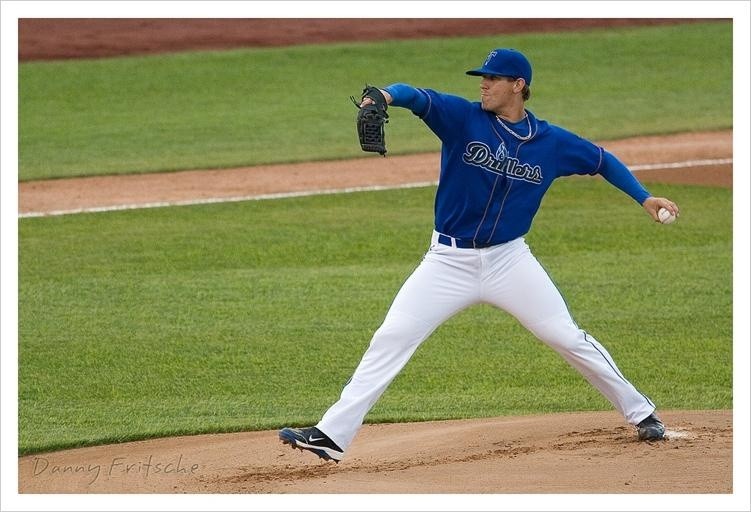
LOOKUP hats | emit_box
[465,48,533,85]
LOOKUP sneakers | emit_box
[635,412,665,441]
[279,426,344,464]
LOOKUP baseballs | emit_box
[658,208,676,225]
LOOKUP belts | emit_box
[438,235,490,249]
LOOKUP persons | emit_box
[276,47,682,465]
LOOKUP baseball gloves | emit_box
[349,85,389,157]
